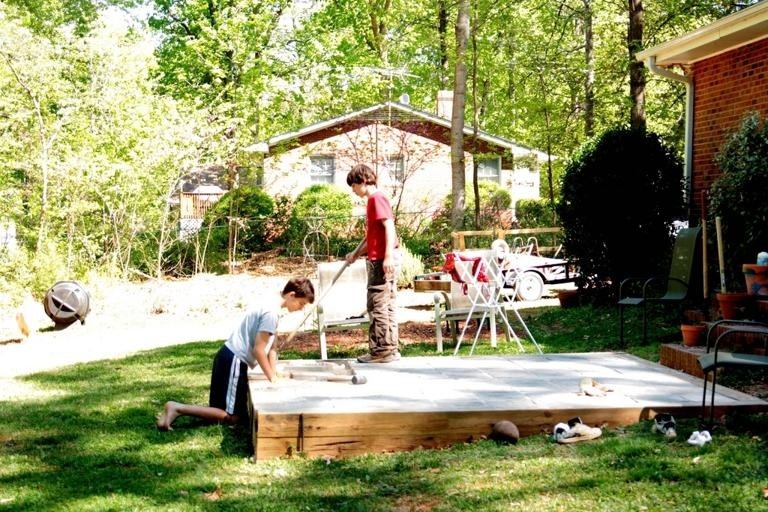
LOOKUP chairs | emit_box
[433,248,511,355]
[618,227,703,347]
[696,320,768,425]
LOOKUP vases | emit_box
[680,263,768,346]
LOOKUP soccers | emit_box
[492,420,519,445]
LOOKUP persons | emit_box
[157,277,314,431]
[345,164,400,363]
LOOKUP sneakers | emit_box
[357,352,401,363]
[652,413,677,438]
[556,417,602,443]
